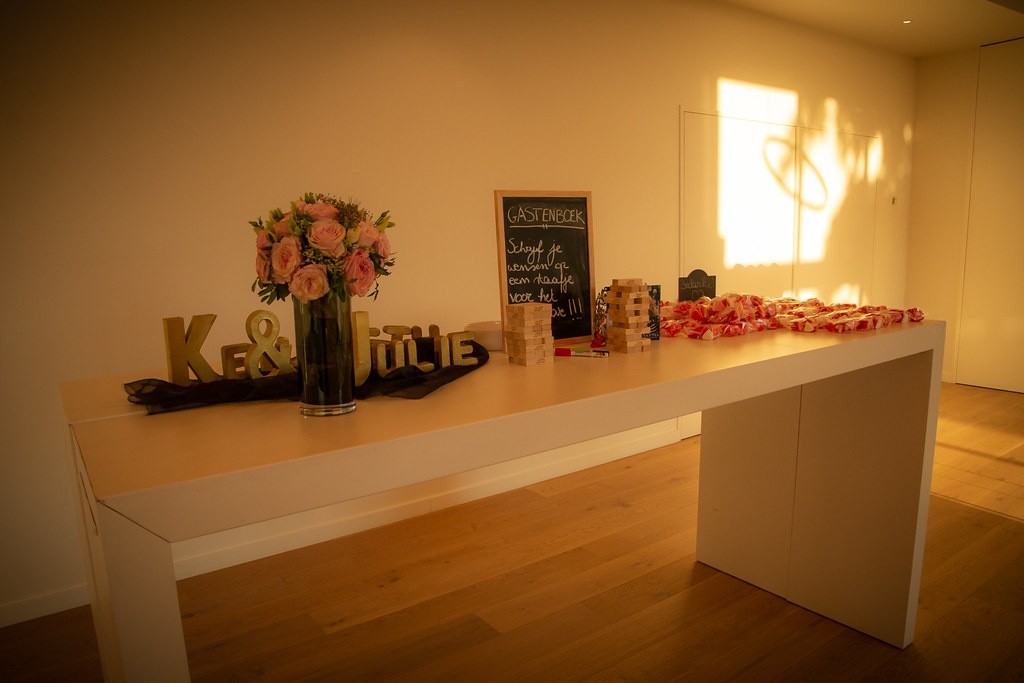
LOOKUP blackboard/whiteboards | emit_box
[492,188,594,347]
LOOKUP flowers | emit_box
[251,191,395,305]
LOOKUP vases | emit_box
[292,291,356,416]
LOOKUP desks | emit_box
[58,319,947,683]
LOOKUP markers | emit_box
[555,347,609,358]
